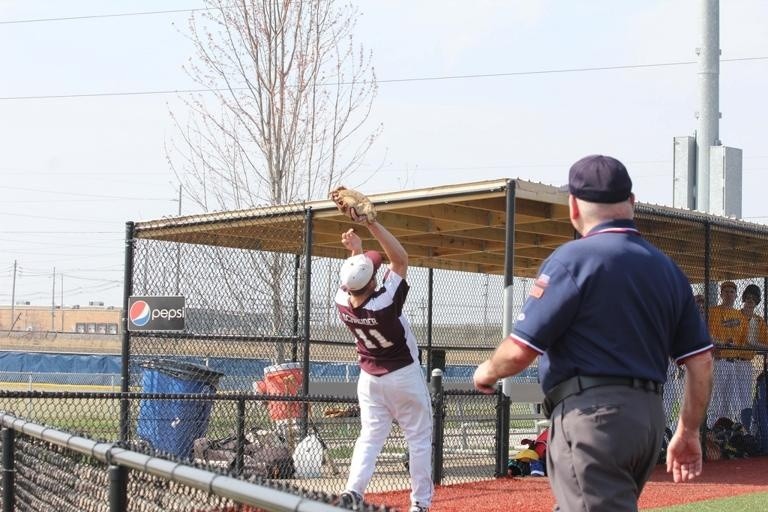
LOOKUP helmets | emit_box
[515,449,539,461]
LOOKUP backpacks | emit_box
[657,417,759,465]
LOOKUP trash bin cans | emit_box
[136,358,224,455]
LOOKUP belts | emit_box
[542,374,664,420]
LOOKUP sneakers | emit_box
[410,501,429,512]
[341,489,364,511]
[529,461,545,476]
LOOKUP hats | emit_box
[694,281,762,306]
[568,154,631,203]
[339,250,381,291]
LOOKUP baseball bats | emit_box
[310,419,340,477]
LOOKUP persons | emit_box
[333,208,435,512]
[663,354,676,431]
[672,289,705,436]
[473,156,715,511]
[707,282,752,431]
[734,284,768,424]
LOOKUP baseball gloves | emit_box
[332,186,377,224]
